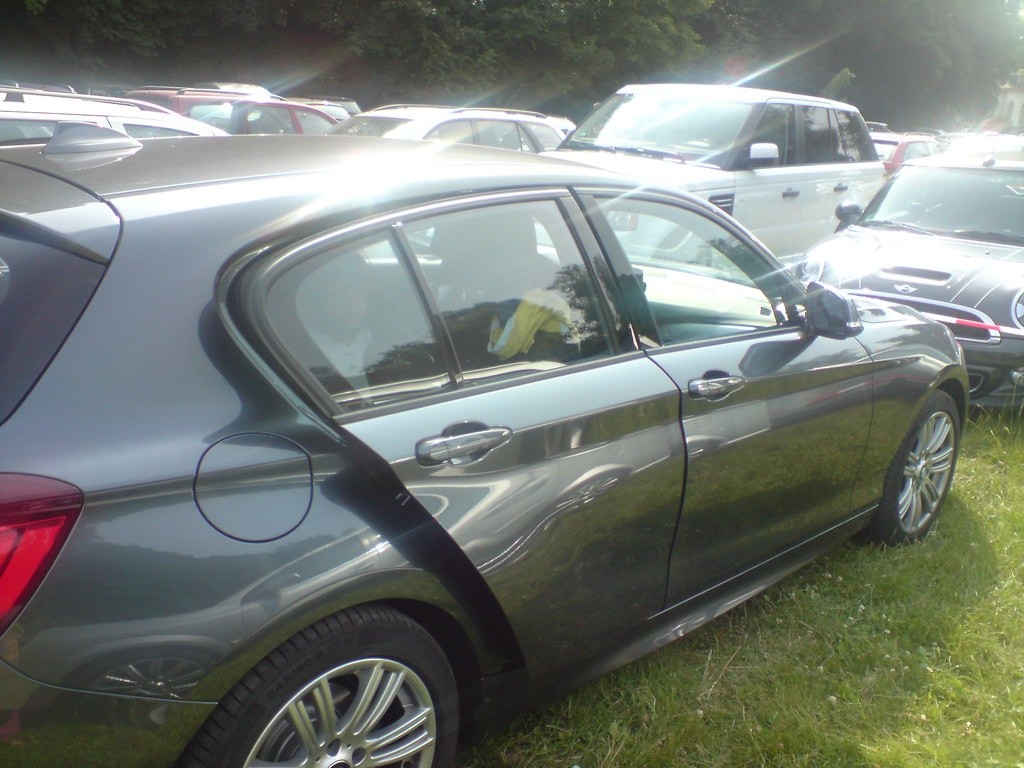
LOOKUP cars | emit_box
[2,121,971,767]
[284,95,367,134]
[867,122,1024,175]
[786,156,1023,419]
[534,83,886,280]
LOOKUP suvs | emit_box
[124,86,338,135]
[0,87,231,146]
[324,103,567,261]
